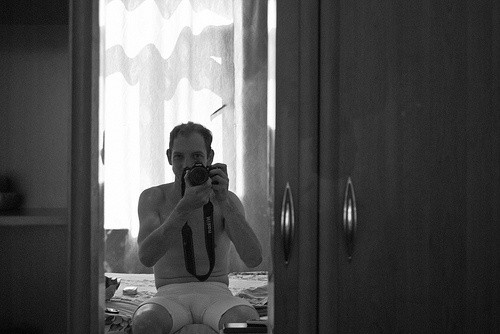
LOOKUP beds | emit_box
[105,272,268,334]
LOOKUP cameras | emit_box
[181,163,215,186]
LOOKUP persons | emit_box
[130,123,263,333]
